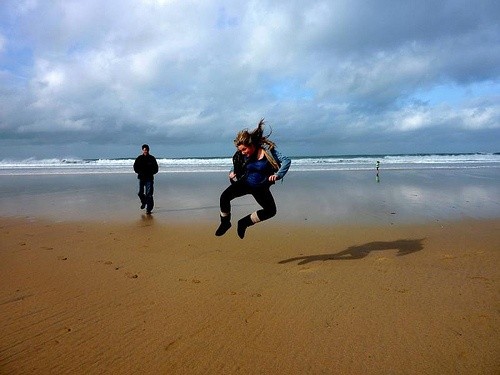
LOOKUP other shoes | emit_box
[141,203,146,209]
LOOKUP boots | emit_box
[215,213,232,236]
[237,213,255,239]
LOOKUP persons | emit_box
[376,161,380,170]
[215,118,292,239]
[133,144,158,214]
